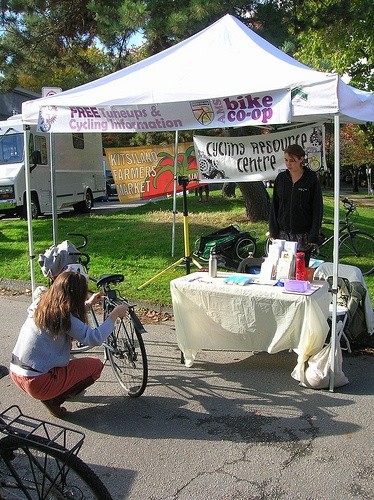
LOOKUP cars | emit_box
[105,170,118,195]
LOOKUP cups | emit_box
[305,268,314,282]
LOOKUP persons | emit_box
[269,143,323,268]
[8,270,127,418]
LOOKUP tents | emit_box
[21,12,374,391]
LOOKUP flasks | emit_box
[209,252,217,278]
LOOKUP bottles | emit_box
[296,252,305,280]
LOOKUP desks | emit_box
[170,271,328,384]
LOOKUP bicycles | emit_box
[66,233,148,398]
[264,195,374,276]
[0,365,113,500]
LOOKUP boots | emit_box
[41,376,94,418]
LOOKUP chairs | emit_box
[288,262,361,353]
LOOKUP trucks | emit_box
[0,114,106,221]
